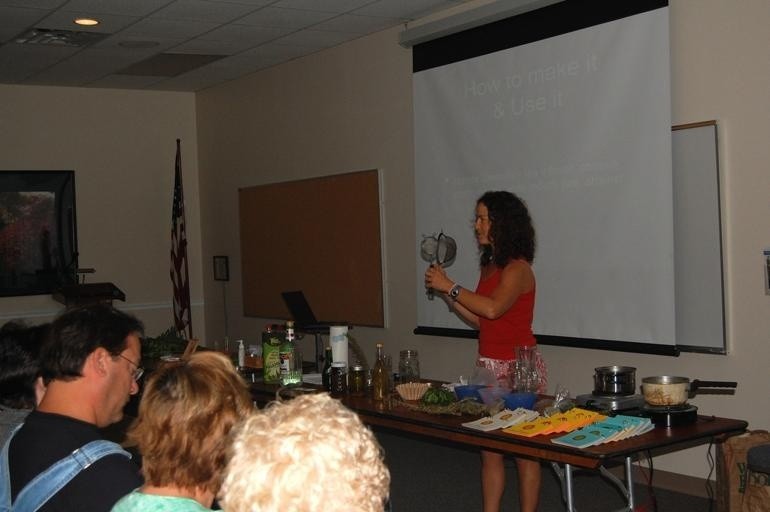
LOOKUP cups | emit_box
[290,348,303,385]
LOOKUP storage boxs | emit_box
[715,429,770,512]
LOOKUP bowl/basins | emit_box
[396,382,429,401]
[503,393,538,410]
[477,387,513,406]
[455,385,486,401]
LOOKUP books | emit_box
[426,191,547,512]
[461,407,656,450]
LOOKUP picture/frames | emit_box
[213,256,229,280]
[0,170,79,297]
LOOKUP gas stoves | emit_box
[643,405,699,426]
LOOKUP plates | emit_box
[302,373,323,385]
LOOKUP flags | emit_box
[170,139,193,339]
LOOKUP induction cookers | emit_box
[575,393,642,427]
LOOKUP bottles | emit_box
[373,344,389,399]
[322,347,334,389]
[329,362,348,397]
[278,321,297,385]
[262,323,282,384]
[349,364,366,396]
[513,345,537,392]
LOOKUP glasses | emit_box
[119,353,145,382]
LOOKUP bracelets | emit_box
[441,293,456,312]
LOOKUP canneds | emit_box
[348,366,365,393]
[331,362,348,399]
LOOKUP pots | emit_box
[594,364,637,395]
[641,375,737,404]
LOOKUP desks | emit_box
[132,339,748,512]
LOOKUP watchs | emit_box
[450,285,462,299]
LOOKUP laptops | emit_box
[281,289,353,331]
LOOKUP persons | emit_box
[217,391,391,512]
[0,319,52,450]
[0,304,144,512]
[109,351,253,512]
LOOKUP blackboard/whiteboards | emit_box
[237,169,392,330]
[671,121,729,355]
